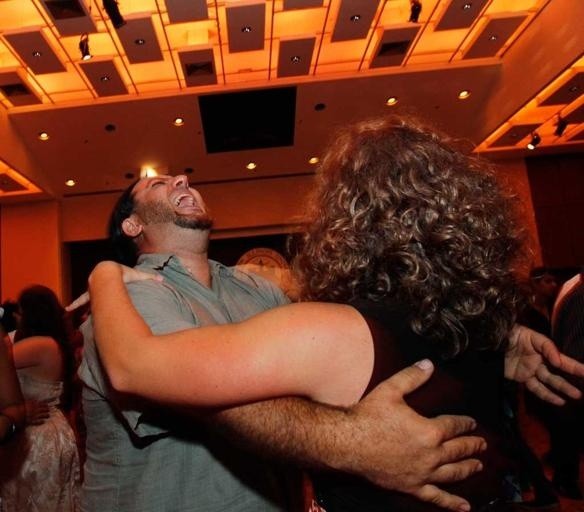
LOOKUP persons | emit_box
[74,175,584,511]
[0,284,91,512]
[65,113,561,512]
[517,259,584,499]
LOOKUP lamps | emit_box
[527,131,541,150]
[78,32,92,60]
[409,2,421,23]
